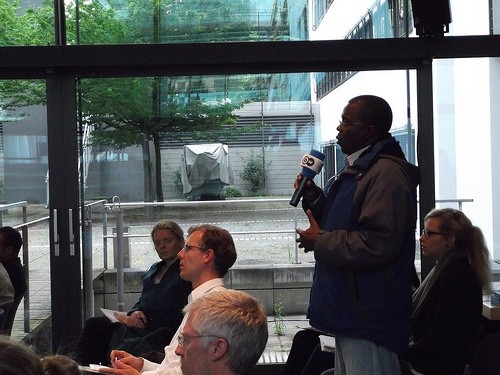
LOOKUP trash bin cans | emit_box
[112,224,130,268]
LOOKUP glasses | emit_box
[178,333,230,350]
[182,243,209,254]
[422,229,445,238]
[339,118,368,128]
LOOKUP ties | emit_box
[323,157,350,195]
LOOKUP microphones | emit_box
[289,148,326,208]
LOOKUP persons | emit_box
[0,263,15,313]
[0,334,45,375]
[175,289,269,375]
[41,355,81,375]
[281,207,494,375]
[293,96,421,375]
[76,220,193,366]
[0,226,27,330]
[99,225,237,375]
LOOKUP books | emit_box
[100,308,128,323]
[319,335,335,352]
